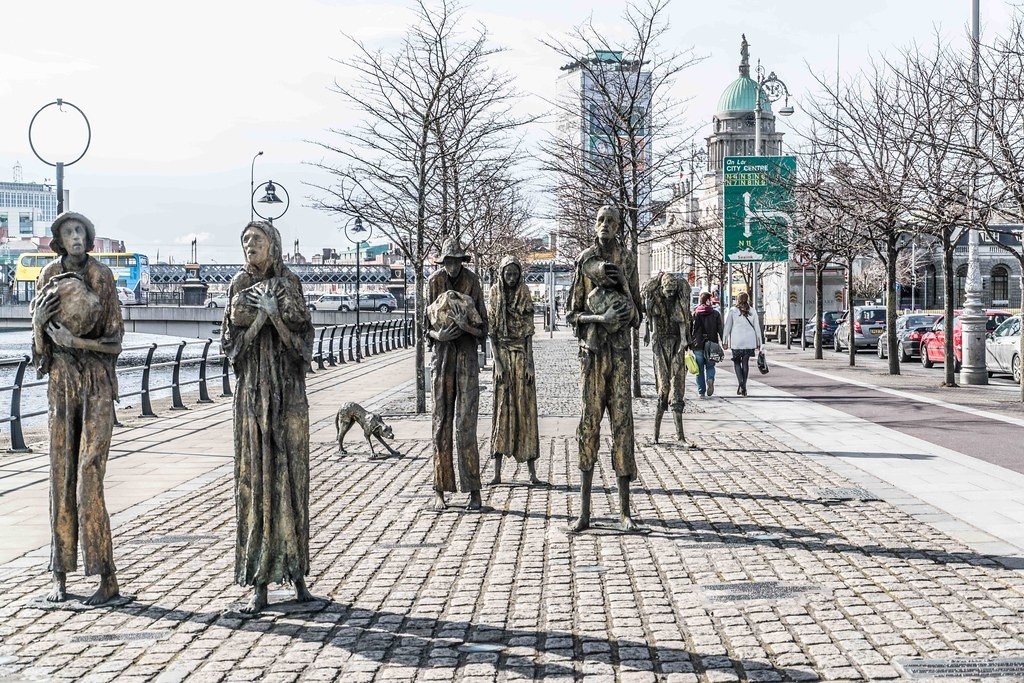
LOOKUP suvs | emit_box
[305,293,356,312]
[351,292,398,313]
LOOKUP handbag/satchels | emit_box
[684,348,700,375]
[757,351,769,374]
[703,339,725,364]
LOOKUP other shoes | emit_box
[707,379,714,396]
[700,393,706,399]
[737,383,748,397]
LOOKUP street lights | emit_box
[250,151,290,224]
[752,60,796,344]
[394,234,416,349]
[345,215,373,363]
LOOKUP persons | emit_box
[220,221,315,615]
[689,292,724,397]
[30,211,124,605]
[638,273,693,447]
[486,256,540,486]
[565,206,638,531]
[723,292,762,396]
[423,238,490,510]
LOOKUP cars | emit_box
[801,310,846,348]
[984,313,1023,384]
[834,305,886,354]
[877,313,942,364]
[918,308,1013,373]
[115,286,136,305]
[203,293,227,309]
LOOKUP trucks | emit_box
[760,261,847,344]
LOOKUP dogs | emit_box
[335,402,399,458]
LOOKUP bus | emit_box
[12,253,150,305]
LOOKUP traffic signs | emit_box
[722,156,795,263]
